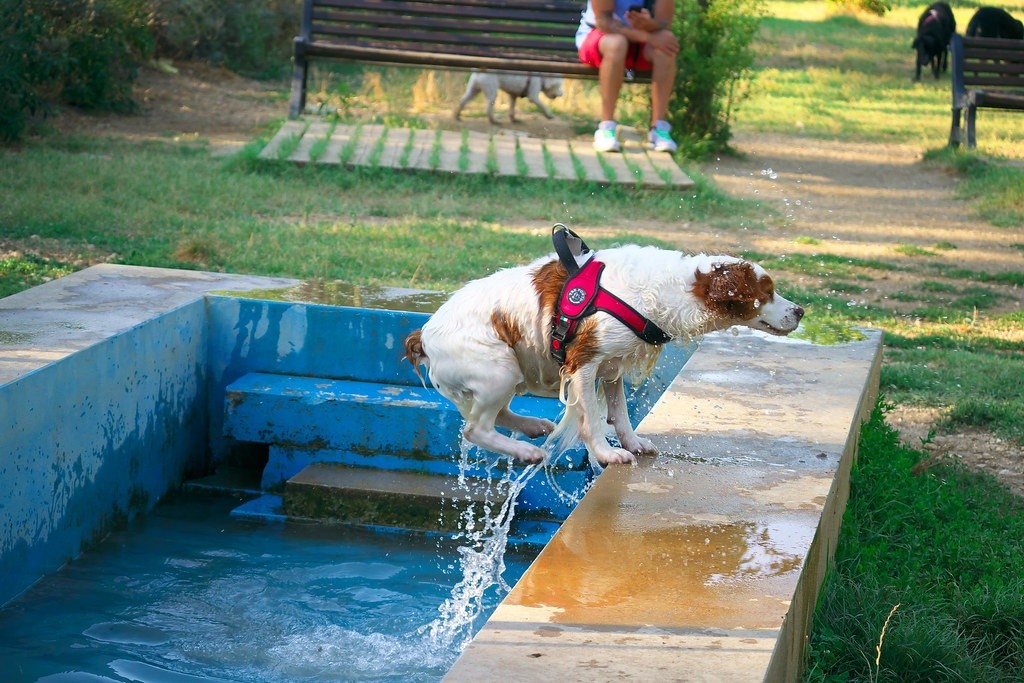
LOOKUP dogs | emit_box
[453,67,564,125]
[910,2,956,82]
[965,6,1024,77]
[404,242,804,466]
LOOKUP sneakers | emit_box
[648,119,677,153]
[594,120,619,152]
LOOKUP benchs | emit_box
[947,33,1024,148]
[288,0,655,121]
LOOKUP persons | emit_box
[574,0,678,152]
